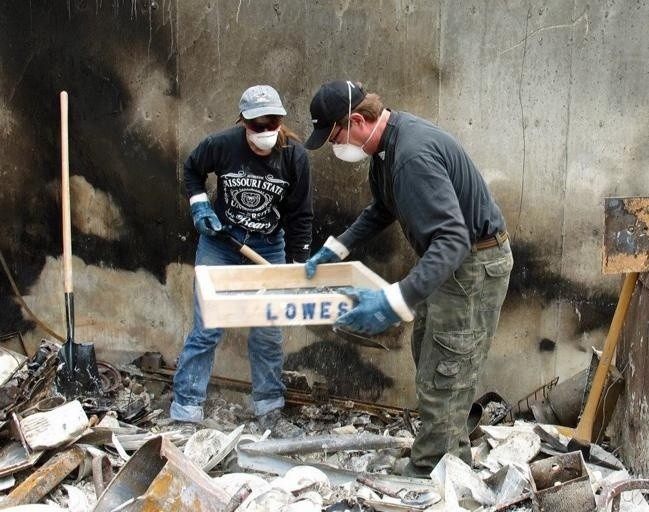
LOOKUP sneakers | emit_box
[258,407,306,439]
[170,420,197,440]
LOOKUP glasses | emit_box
[329,125,346,144]
[240,117,281,133]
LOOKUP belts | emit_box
[470,229,507,252]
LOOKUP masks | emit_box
[246,128,280,152]
[331,144,368,163]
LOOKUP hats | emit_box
[303,81,367,151]
[238,84,288,120]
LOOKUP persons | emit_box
[171,85,316,434]
[304,80,514,477]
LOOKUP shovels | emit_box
[51,91,102,406]
[204,218,389,351]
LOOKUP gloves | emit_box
[188,191,222,239]
[306,236,351,279]
[332,281,415,337]
